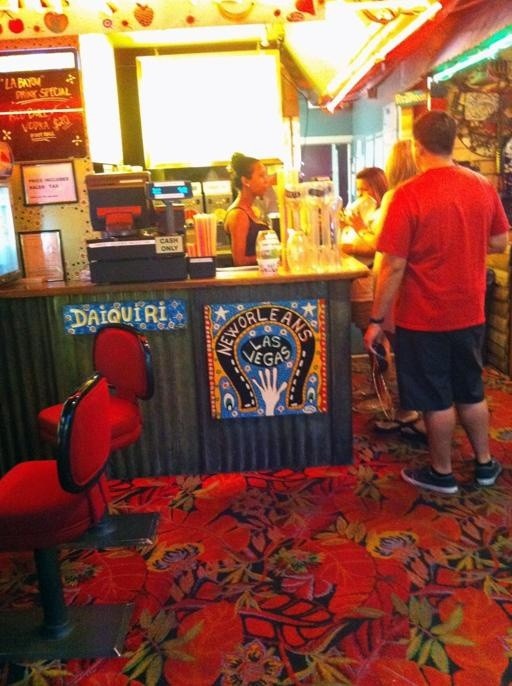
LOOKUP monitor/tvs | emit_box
[86,171,156,240]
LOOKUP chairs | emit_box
[0,371,135,660]
[37,323,160,549]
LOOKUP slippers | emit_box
[368,417,427,444]
[352,399,391,413]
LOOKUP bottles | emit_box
[284,182,337,255]
[345,193,376,221]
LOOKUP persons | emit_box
[224,152,270,267]
[365,111,508,492]
[341,139,427,437]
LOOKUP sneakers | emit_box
[401,465,458,494]
[475,460,502,485]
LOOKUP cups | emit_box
[253,227,311,272]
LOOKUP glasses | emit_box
[373,344,387,375]
